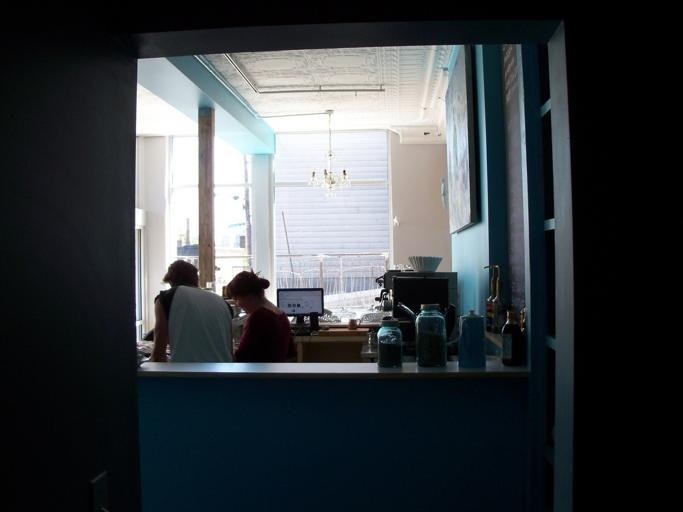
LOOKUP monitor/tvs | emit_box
[277,288,324,330]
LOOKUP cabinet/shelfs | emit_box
[538,99,559,352]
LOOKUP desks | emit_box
[293,326,370,363]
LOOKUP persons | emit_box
[148,259,234,363]
[224,270,298,362]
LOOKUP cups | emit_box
[348,318,361,330]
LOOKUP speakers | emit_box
[309,312,319,331]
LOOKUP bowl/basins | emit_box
[408,255,444,272]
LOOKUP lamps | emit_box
[306,107,352,197]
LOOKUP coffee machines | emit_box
[374,270,462,357]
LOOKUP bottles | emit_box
[458,310,487,370]
[376,319,404,369]
[415,302,449,368]
[483,265,502,333]
[500,306,526,365]
